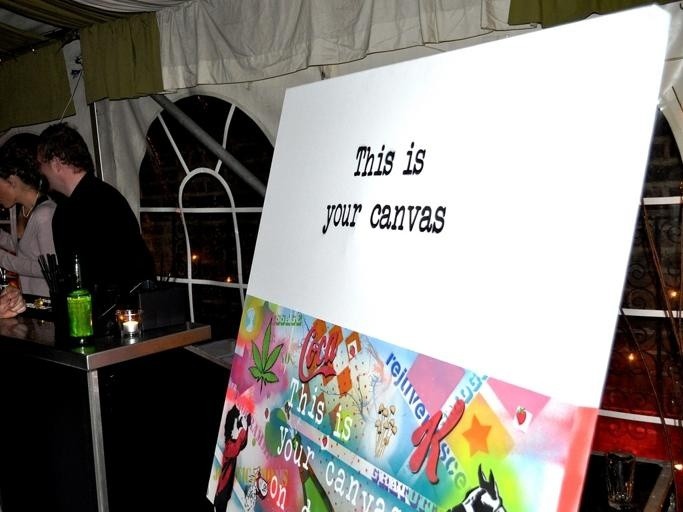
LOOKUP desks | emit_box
[0,306,214,512]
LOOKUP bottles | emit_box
[65,254,94,337]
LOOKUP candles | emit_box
[121,321,138,334]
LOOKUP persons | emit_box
[213,404,253,512]
[35,122,159,324]
[1,318,29,340]
[0,285,27,319]
[0,158,57,298]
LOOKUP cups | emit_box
[605,451,636,509]
[116,309,144,341]
